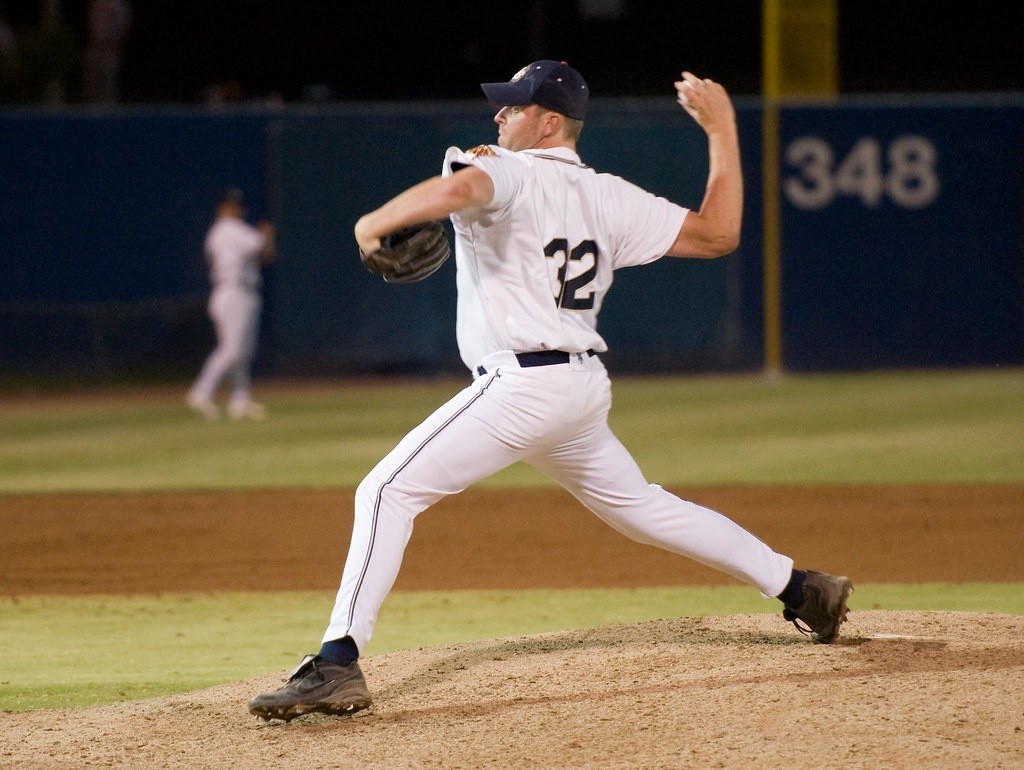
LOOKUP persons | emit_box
[247,58,854,717]
[188,190,272,417]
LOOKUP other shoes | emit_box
[226,399,270,424]
[181,389,222,422]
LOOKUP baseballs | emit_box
[677,79,698,110]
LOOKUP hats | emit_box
[480,60,590,120]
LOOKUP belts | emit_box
[476,346,597,376]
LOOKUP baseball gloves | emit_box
[359,219,453,284]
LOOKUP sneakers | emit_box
[783,569,855,644]
[246,652,373,723]
